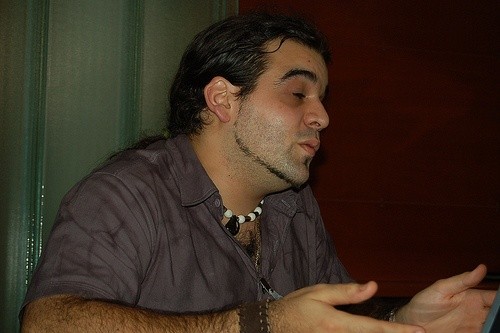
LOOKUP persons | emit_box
[18,16,497,333]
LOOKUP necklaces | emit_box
[219,198,265,236]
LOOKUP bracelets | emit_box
[239,297,270,333]
